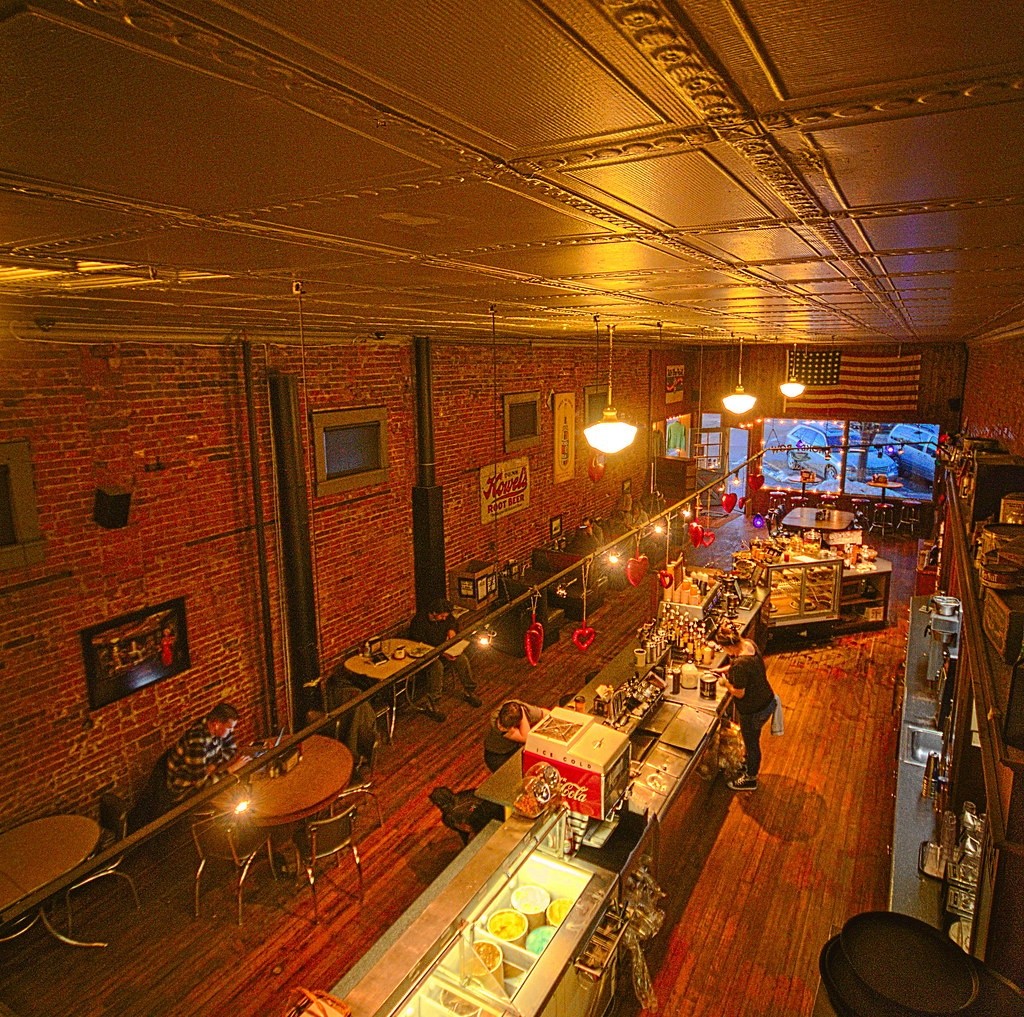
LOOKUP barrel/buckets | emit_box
[461,942,505,995]
[487,911,529,979]
[546,898,574,928]
[440,979,486,1017]
[526,927,557,958]
[511,887,550,929]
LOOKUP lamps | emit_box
[723,337,757,414]
[780,343,805,398]
[583,324,637,455]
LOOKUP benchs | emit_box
[474,498,693,658]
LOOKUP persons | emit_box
[118,650,131,665]
[167,704,252,804]
[625,504,648,530]
[712,625,777,790]
[484,700,551,771]
[408,599,482,722]
[162,630,173,669]
[577,517,604,548]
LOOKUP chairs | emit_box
[766,504,794,537]
[848,511,864,529]
[195,660,456,927]
[51,792,142,940]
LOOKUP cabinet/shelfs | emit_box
[832,557,893,636]
[935,463,1024,986]
[658,455,697,507]
[732,551,844,645]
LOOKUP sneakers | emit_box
[728,773,757,790]
[725,765,747,777]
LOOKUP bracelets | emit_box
[722,682,725,686]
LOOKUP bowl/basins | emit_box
[934,595,959,616]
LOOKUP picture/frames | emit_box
[549,514,562,539]
[458,577,476,598]
[82,596,191,709]
[476,572,497,603]
[623,479,632,494]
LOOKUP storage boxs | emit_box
[983,588,1023,663]
[1000,493,1023,525]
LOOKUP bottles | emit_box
[673,667,680,693]
[667,669,672,692]
[681,661,698,688]
[660,599,706,661]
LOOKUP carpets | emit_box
[698,505,741,528]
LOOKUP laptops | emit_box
[227,726,289,773]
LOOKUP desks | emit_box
[789,476,821,497]
[642,530,651,538]
[344,638,439,740]
[210,735,355,848]
[0,813,108,948]
[782,507,855,535]
[511,570,578,592]
[669,513,678,520]
[867,481,903,503]
[915,538,937,596]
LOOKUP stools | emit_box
[769,492,787,509]
[869,503,895,535]
[821,495,839,507]
[897,499,921,532]
[790,497,808,509]
[559,670,601,709]
[851,499,870,527]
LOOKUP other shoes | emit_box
[426,704,447,721]
[463,691,482,707]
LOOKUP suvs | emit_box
[785,421,899,485]
[837,421,940,492]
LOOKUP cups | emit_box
[922,749,985,852]
[663,561,698,605]
